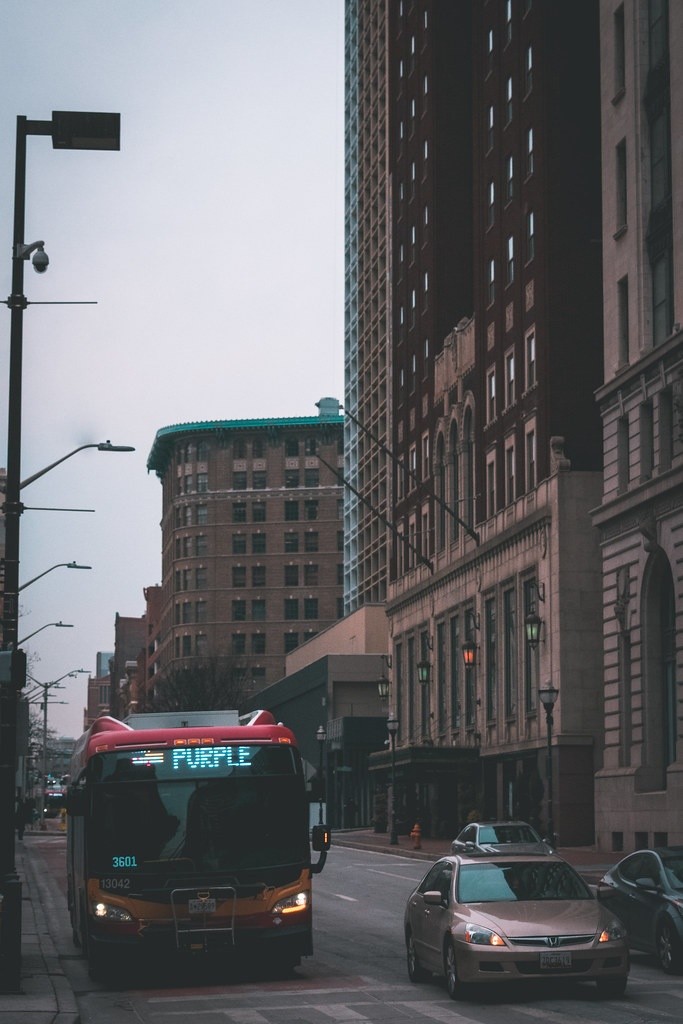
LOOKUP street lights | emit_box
[386,718,400,846]
[314,725,328,824]
[1,108,123,1001]
[40,668,94,830]
[537,687,559,847]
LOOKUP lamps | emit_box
[524,610,545,650]
[377,674,392,700]
[462,637,480,669]
[417,658,431,688]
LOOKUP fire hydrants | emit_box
[411,823,423,850]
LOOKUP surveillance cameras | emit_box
[32,252,49,274]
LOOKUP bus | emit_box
[44,790,65,818]
[66,717,334,978]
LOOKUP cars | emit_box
[60,774,71,786]
[46,777,57,786]
[405,853,630,997]
[450,820,555,861]
[597,844,683,977]
